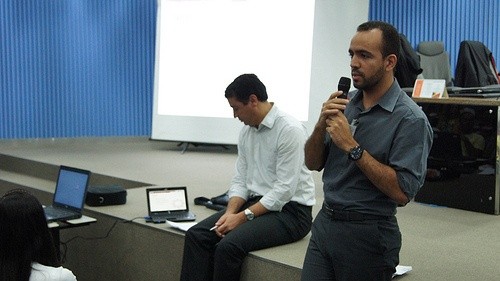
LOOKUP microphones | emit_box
[335,77,351,113]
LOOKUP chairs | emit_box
[417,42,499,171]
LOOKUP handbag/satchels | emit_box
[211,189,229,205]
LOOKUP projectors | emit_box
[86,184,127,208]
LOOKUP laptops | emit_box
[43,165,90,223]
[146,186,196,223]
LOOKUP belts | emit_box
[321,203,382,221]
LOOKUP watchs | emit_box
[348,145,365,160]
[244,208,254,221]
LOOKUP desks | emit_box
[408,96,499,170]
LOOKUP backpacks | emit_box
[394,33,421,87]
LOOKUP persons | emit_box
[455,109,485,160]
[180,74,315,281]
[0,188,78,281]
[301,21,434,281]
